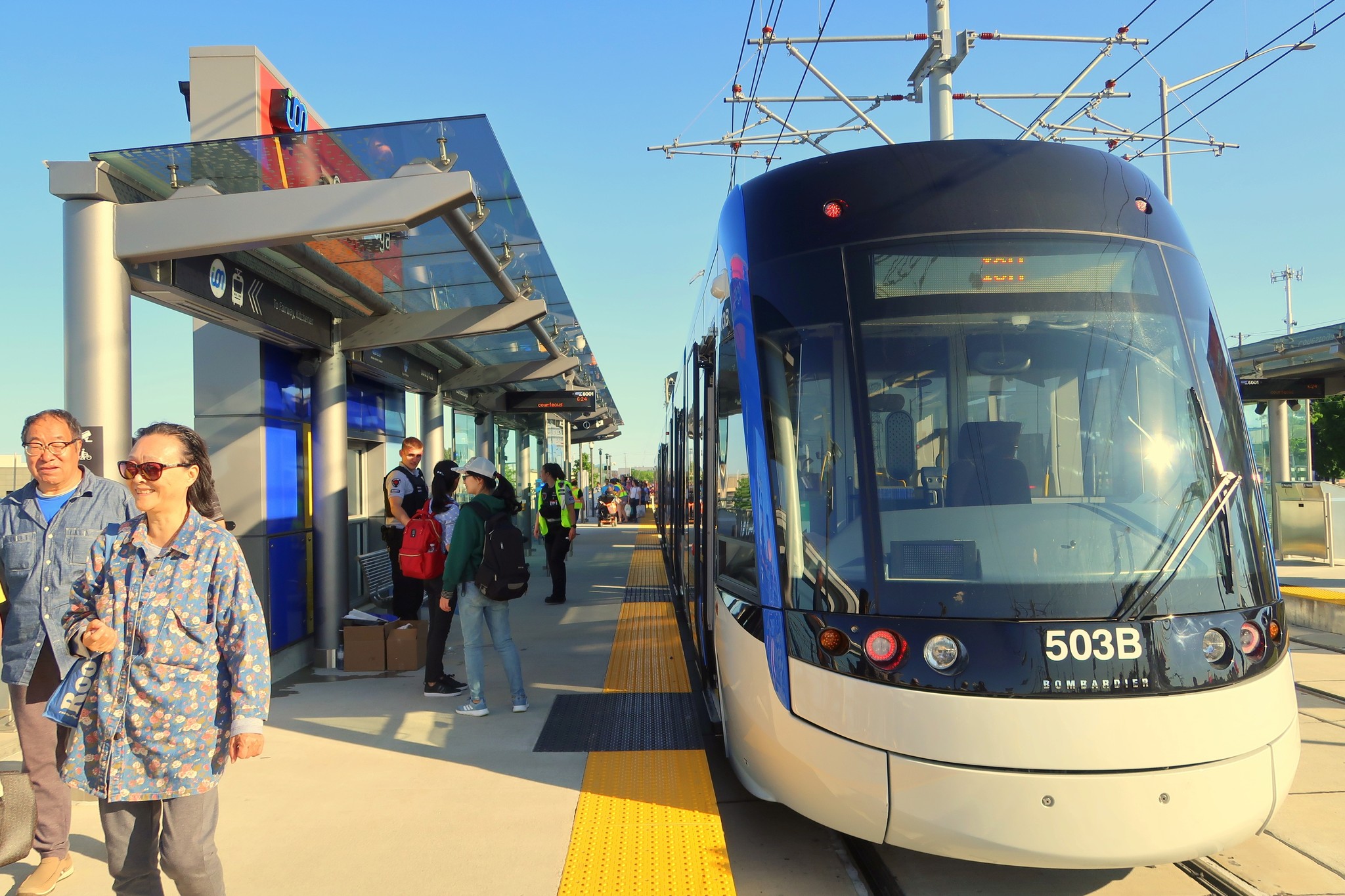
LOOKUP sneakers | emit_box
[440,674,468,690]
[511,695,529,712]
[422,681,462,697]
[455,696,489,717]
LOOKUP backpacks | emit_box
[399,499,447,579]
[465,499,530,602]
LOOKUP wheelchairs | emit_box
[596,494,618,527]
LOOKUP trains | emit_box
[651,137,1307,873]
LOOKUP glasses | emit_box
[21,438,80,455]
[626,482,630,483]
[462,475,473,480]
[117,459,190,481]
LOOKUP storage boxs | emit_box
[344,617,428,671]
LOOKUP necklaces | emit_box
[148,507,189,547]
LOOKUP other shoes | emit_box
[545,593,566,605]
[622,521,627,523]
[634,522,638,523]
[16,856,74,895]
[617,522,622,524]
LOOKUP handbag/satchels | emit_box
[630,498,635,503]
[636,505,645,518]
[44,522,124,728]
[618,496,628,504]
[645,492,649,501]
[0,770,38,868]
[600,498,617,514]
[624,504,631,516]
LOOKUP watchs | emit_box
[571,525,577,528]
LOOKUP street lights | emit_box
[608,455,612,480]
[598,448,604,488]
[589,442,595,517]
[605,453,609,480]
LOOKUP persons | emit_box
[610,475,654,518]
[607,486,612,495]
[610,479,628,524]
[382,437,428,620]
[571,479,586,535]
[439,457,530,716]
[601,479,612,494]
[627,480,641,522]
[593,482,602,492]
[534,462,576,604]
[422,459,469,697]
[0,582,6,803]
[1,410,141,895]
[59,421,272,896]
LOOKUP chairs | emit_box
[945,421,1032,508]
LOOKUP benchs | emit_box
[354,548,429,620]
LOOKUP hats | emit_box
[452,456,495,480]
